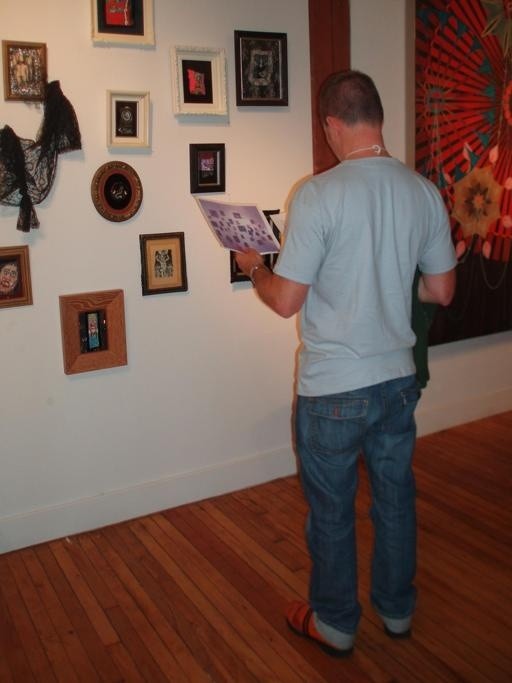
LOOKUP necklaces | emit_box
[342,144,388,159]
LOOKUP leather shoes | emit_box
[286,601,352,656]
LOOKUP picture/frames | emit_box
[234,28,288,106]
[106,88,150,149]
[139,231,188,295]
[3,38,47,100]
[58,288,128,374]
[92,160,143,222]
[171,45,229,117]
[0,244,33,308]
[230,209,283,282]
[91,0,155,47]
[189,142,225,193]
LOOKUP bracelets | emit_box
[249,264,269,287]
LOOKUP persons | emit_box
[235,69,462,662]
